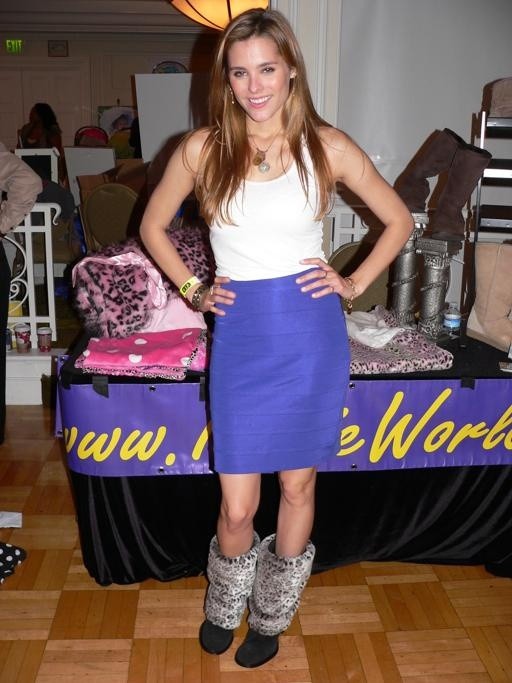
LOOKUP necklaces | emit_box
[245,126,283,172]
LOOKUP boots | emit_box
[234,532,317,669]
[199,529,262,655]
[429,142,493,244]
[464,241,512,357]
[393,128,468,214]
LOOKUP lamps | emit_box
[162,0,272,35]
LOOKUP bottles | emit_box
[442,301,461,348]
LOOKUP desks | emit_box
[49,326,512,587]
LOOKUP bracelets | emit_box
[192,283,208,312]
[180,276,200,297]
[342,276,359,316]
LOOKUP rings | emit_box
[323,270,328,278]
[210,284,217,296]
[331,288,335,293]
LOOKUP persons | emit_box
[140,8,414,669]
[16,102,75,224]
[0,143,44,444]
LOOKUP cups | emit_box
[15,326,31,353]
[38,326,53,353]
